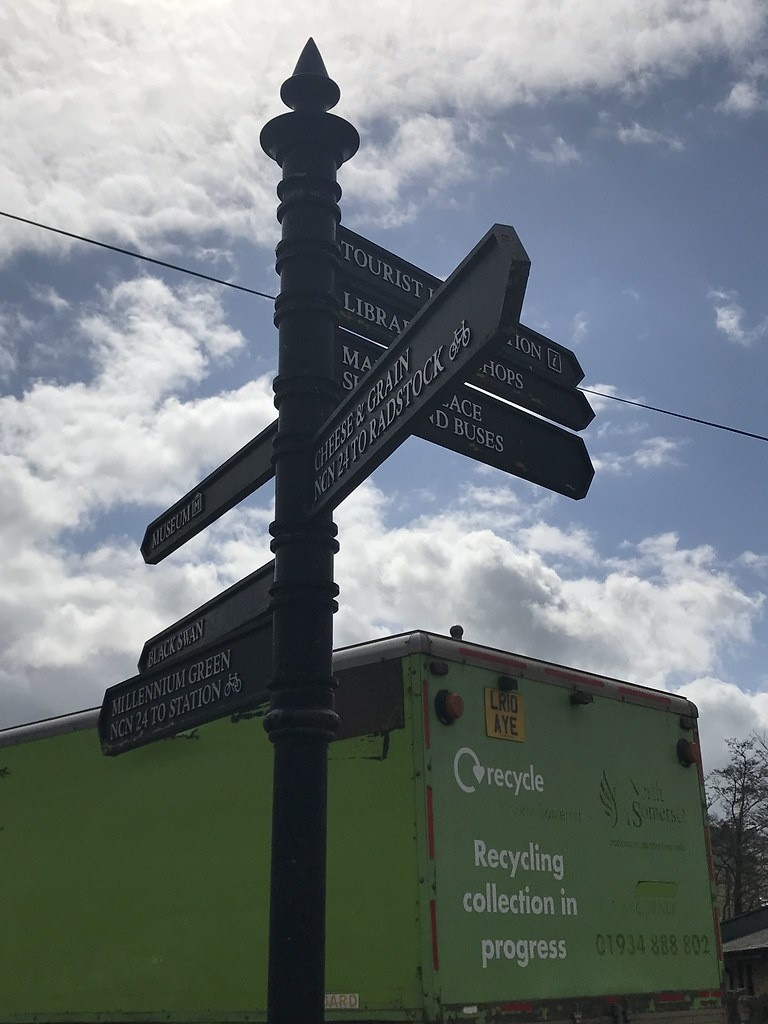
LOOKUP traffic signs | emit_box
[337,223,586,392]
[338,325,597,502]
[99,623,276,759]
[140,419,279,566]
[137,560,279,676]
[307,221,530,524]
[337,282,597,433]
